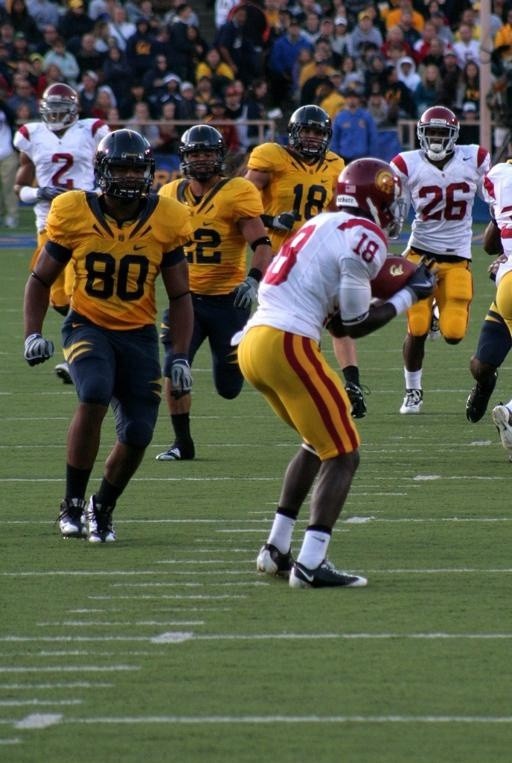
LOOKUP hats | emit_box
[13,0,457,111]
[0,110,7,123]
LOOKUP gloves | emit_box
[406,261,440,302]
[38,184,70,200]
[273,209,297,232]
[23,331,55,368]
[170,352,193,398]
[230,266,262,310]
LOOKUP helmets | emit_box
[335,155,405,241]
[419,106,459,161]
[285,102,333,158]
[41,81,81,128]
[93,127,153,203]
[178,123,226,179]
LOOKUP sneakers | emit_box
[256,543,296,578]
[54,362,73,385]
[492,403,512,462]
[288,559,369,591]
[399,388,424,414]
[343,382,367,418]
[84,494,117,542]
[428,295,441,339]
[3,216,20,230]
[156,438,194,464]
[57,498,88,538]
[465,369,498,424]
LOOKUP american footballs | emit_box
[370,258,418,299]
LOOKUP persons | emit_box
[240,105,367,420]
[12,82,110,385]
[483,159,512,454]
[466,220,512,424]
[235,156,436,590]
[23,129,194,543]
[387,105,490,415]
[155,124,273,461]
[0,1,512,177]
